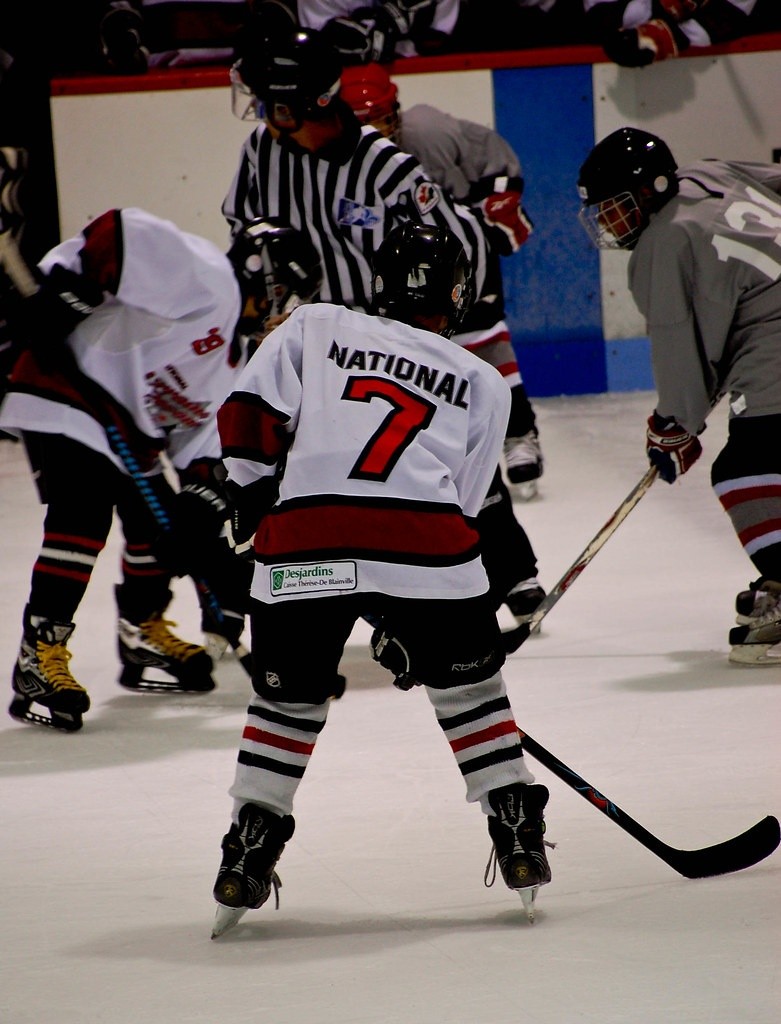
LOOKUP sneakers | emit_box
[7,604,91,733]
[113,582,218,694]
[368,629,389,662]
[503,426,546,500]
[507,576,548,637]
[488,785,551,924]
[729,579,781,665]
[210,802,295,941]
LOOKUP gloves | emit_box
[477,176,535,256]
[17,261,106,351]
[606,19,689,68]
[645,409,701,485]
[161,480,227,569]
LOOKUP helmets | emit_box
[577,125,680,249]
[340,62,399,122]
[371,221,478,338]
[225,217,324,338]
[231,25,342,125]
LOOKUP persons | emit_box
[212,225,557,909]
[340,64,543,484]
[200,18,549,638]
[0,207,325,712]
[0,0,781,445]
[578,122,781,645]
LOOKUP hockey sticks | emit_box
[501,388,730,653]
[359,616,780,880]
[2,227,348,704]
